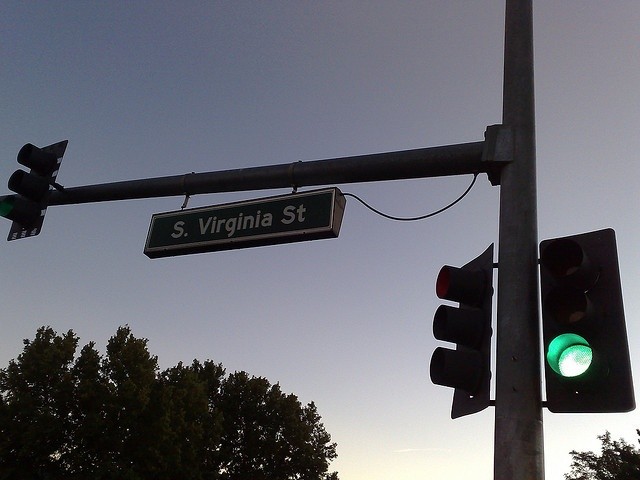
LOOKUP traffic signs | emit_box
[143,187,345,260]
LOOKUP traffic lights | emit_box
[538,227,637,413]
[428,242,495,418]
[0,138,68,242]
